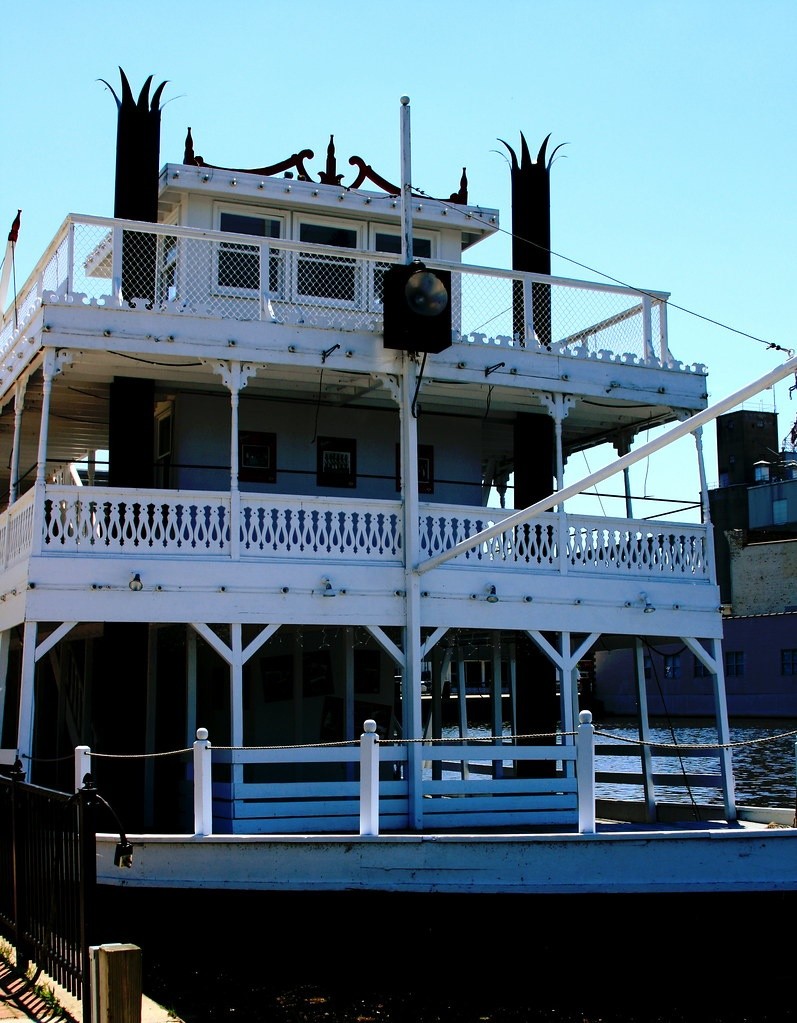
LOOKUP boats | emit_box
[0,64,797,977]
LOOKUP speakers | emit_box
[383,264,451,353]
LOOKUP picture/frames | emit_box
[237,430,277,483]
[316,435,357,488]
[396,442,435,493]
